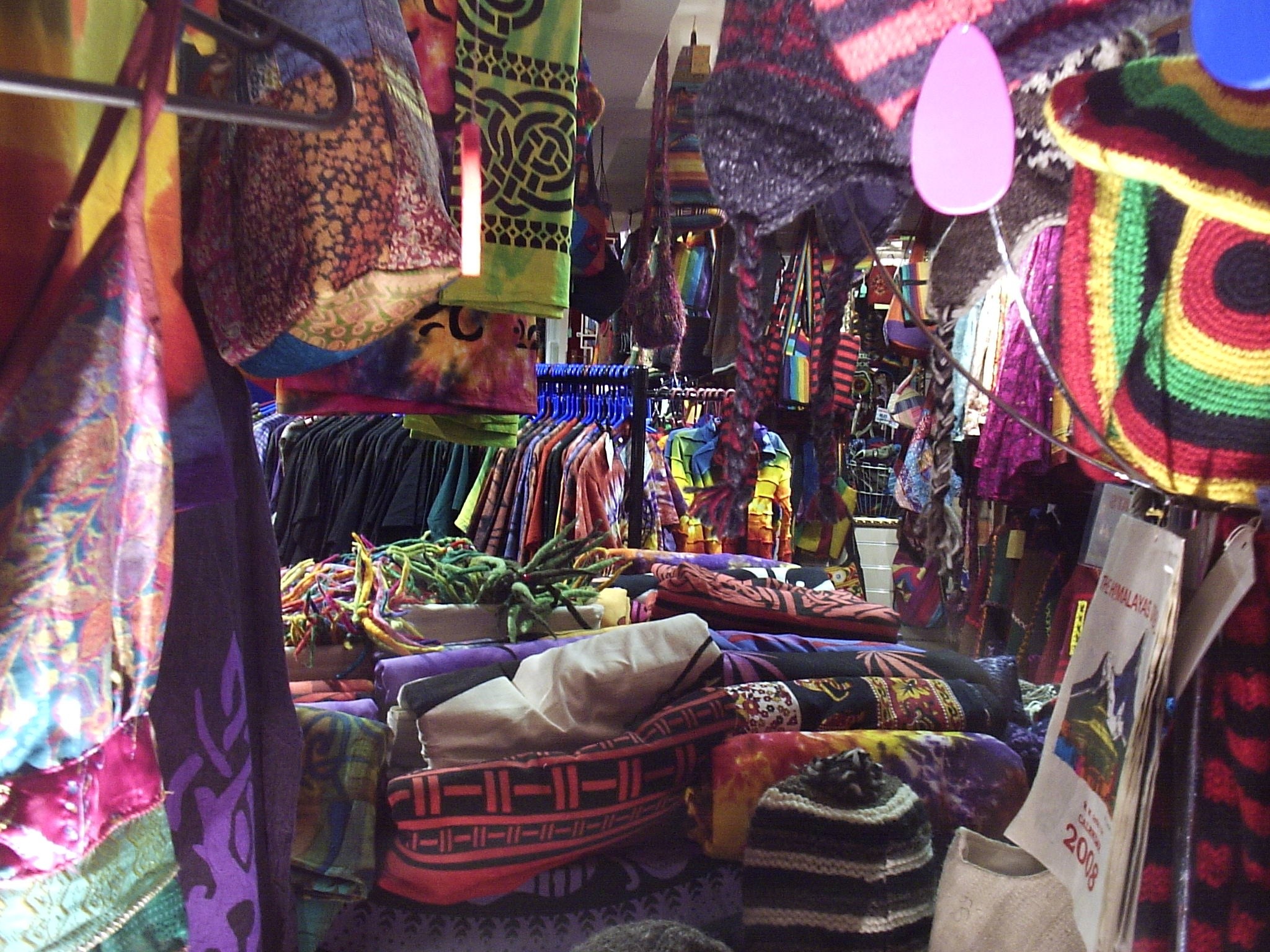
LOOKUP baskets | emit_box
[845,461,904,528]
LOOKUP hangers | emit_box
[530,358,739,434]
[0,0,356,136]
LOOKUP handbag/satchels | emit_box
[621,258,686,349]
[893,408,962,512]
[891,541,948,630]
[2,1,182,776]
[572,200,631,326]
[773,333,860,413]
[886,364,925,430]
[884,281,939,353]
